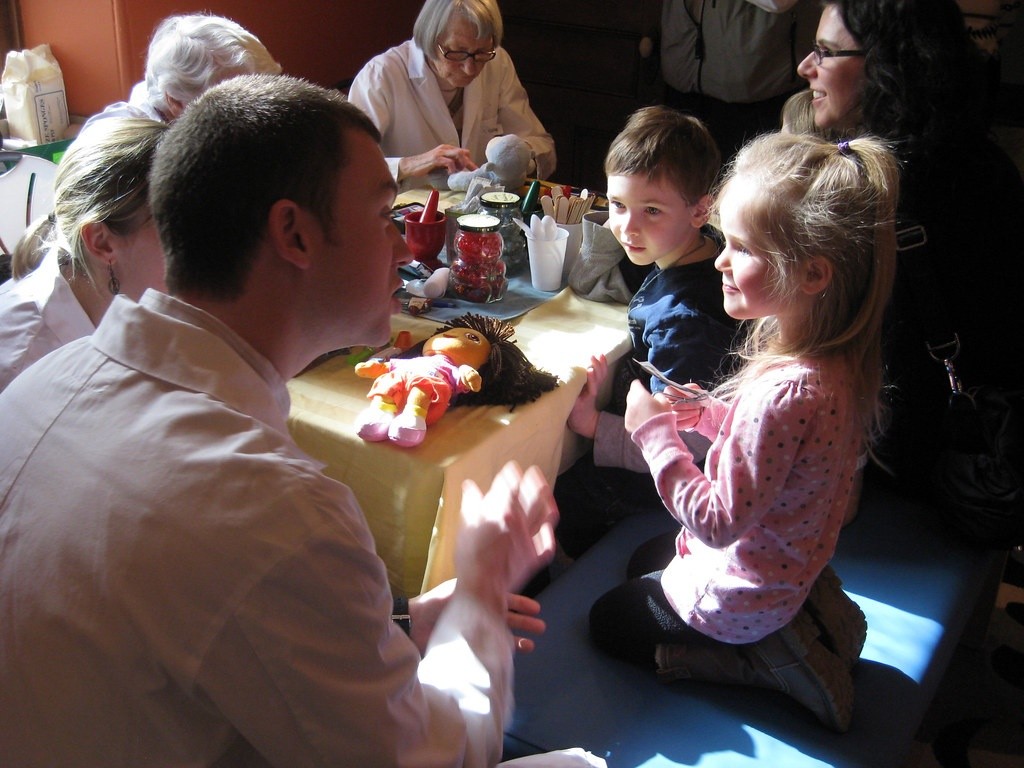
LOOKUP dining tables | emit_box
[287,177,721,598]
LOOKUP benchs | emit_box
[500,480,1012,768]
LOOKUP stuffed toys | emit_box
[446,133,537,193]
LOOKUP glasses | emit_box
[813,40,869,67]
[437,33,497,63]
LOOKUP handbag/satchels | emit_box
[895,217,1024,550]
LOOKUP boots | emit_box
[653,565,867,736]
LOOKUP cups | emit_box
[519,204,544,261]
[405,211,447,269]
[445,209,477,264]
[525,227,569,291]
[557,223,583,272]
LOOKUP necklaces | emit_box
[441,86,458,93]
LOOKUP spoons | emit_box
[513,214,556,241]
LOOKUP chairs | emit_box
[1,150,60,257]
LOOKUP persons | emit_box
[555,104,754,561]
[0,117,170,390]
[0,72,561,768]
[796,0,1024,550]
[347,0,557,189]
[660,0,823,159]
[128,12,283,127]
[353,310,561,448]
[567,129,903,734]
